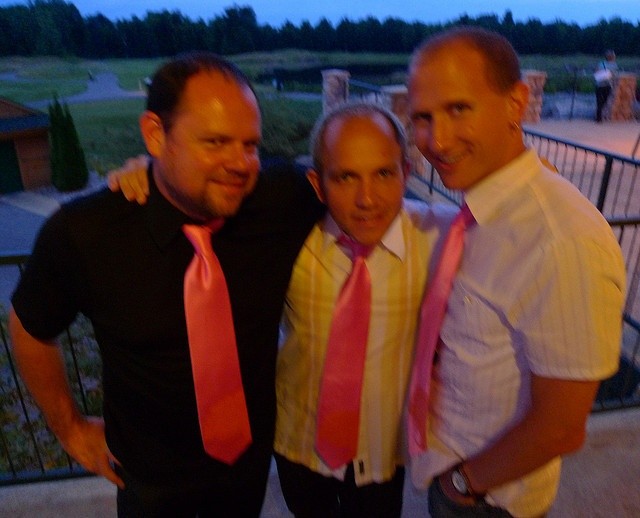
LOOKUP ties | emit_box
[182,223,253,464]
[406,203,473,456]
[315,237,372,472]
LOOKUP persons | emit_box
[8,50,328,518]
[107,102,558,518]
[594,49,620,122]
[400,27,628,518]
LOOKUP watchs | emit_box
[450,463,476,498]
